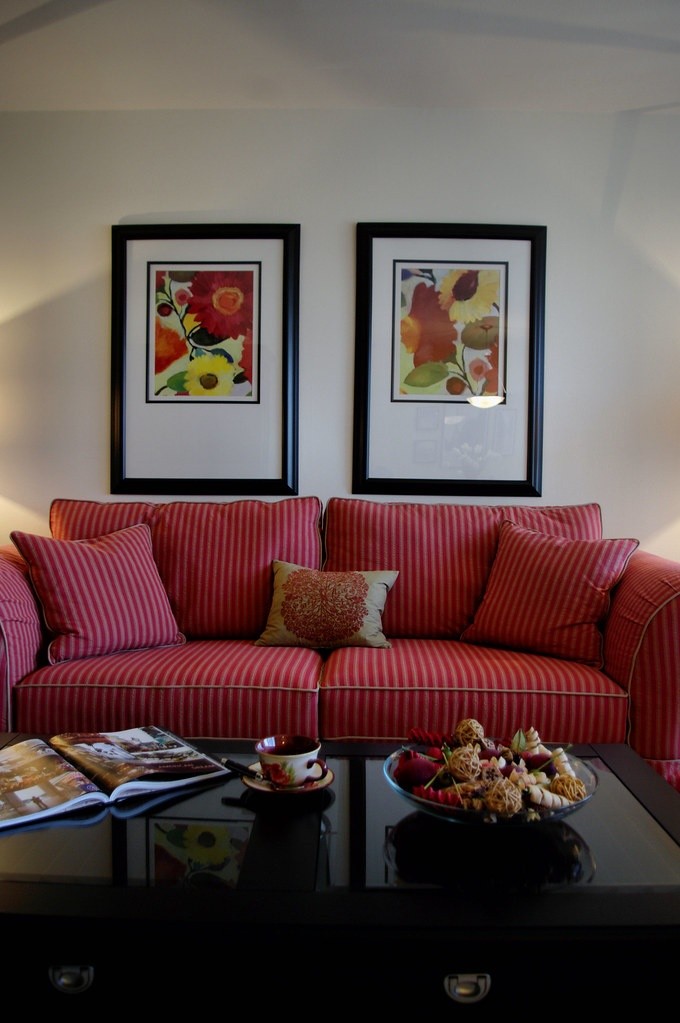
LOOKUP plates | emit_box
[241,761,334,794]
[383,743,600,826]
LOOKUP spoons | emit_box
[221,757,304,790]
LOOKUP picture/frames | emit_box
[352,223,547,497]
[112,757,366,887]
[110,223,301,497]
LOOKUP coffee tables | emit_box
[0,743,680,1023]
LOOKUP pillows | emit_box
[10,525,187,665]
[255,561,403,651]
[458,521,640,671]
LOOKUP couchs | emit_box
[0,545,680,797]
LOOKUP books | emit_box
[0,782,198,836]
[0,726,231,827]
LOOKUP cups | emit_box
[254,735,327,788]
